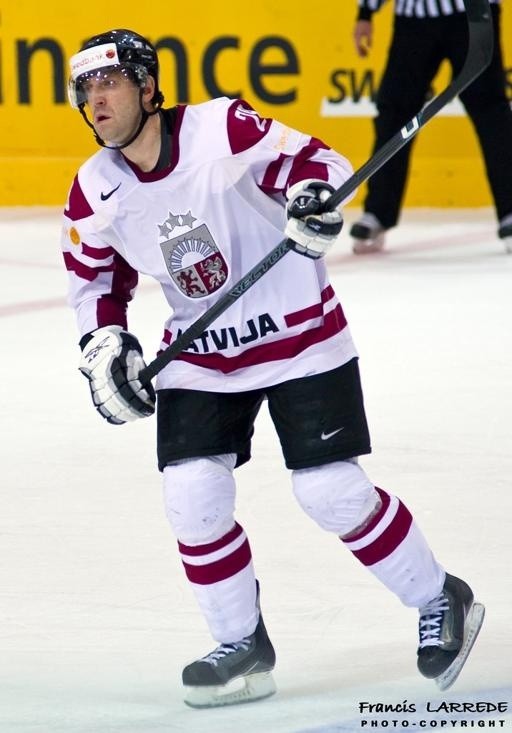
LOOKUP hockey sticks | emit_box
[137,0,494,383]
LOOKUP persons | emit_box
[349,1,512,238]
[60,28,475,688]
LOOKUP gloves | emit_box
[77,329,156,426]
[283,178,344,261]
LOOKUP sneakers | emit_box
[181,613,278,687]
[416,571,475,681]
[498,214,512,239]
[349,212,386,240]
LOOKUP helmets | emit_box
[65,27,164,152]
